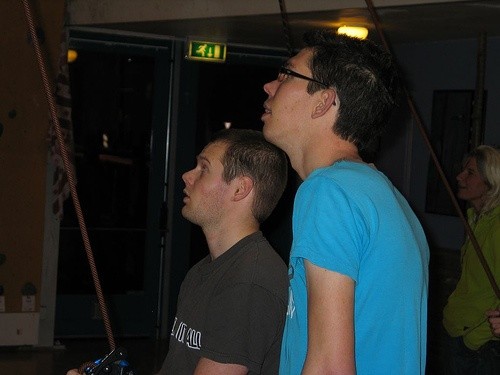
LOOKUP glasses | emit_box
[276,65,337,107]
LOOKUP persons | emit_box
[60,125,293,374]
[251,27,430,374]
[431,143,500,375]
